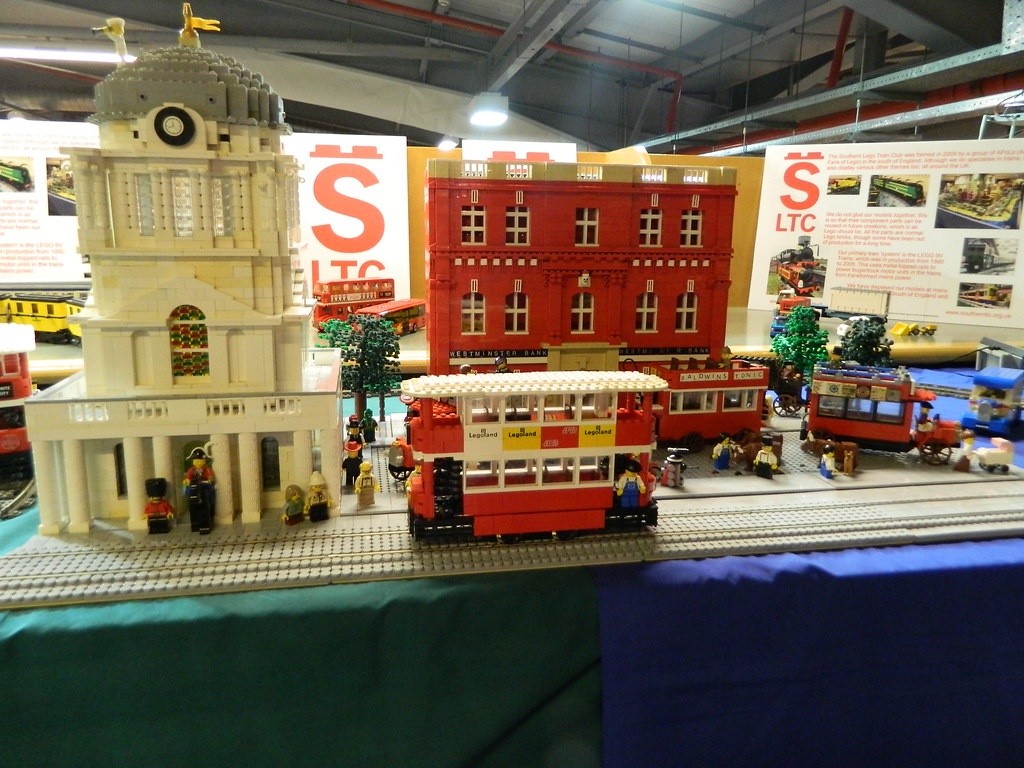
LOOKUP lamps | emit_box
[466,91,509,129]
[435,133,459,153]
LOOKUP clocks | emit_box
[163,116,184,137]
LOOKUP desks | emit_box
[0,367,1023,768]
